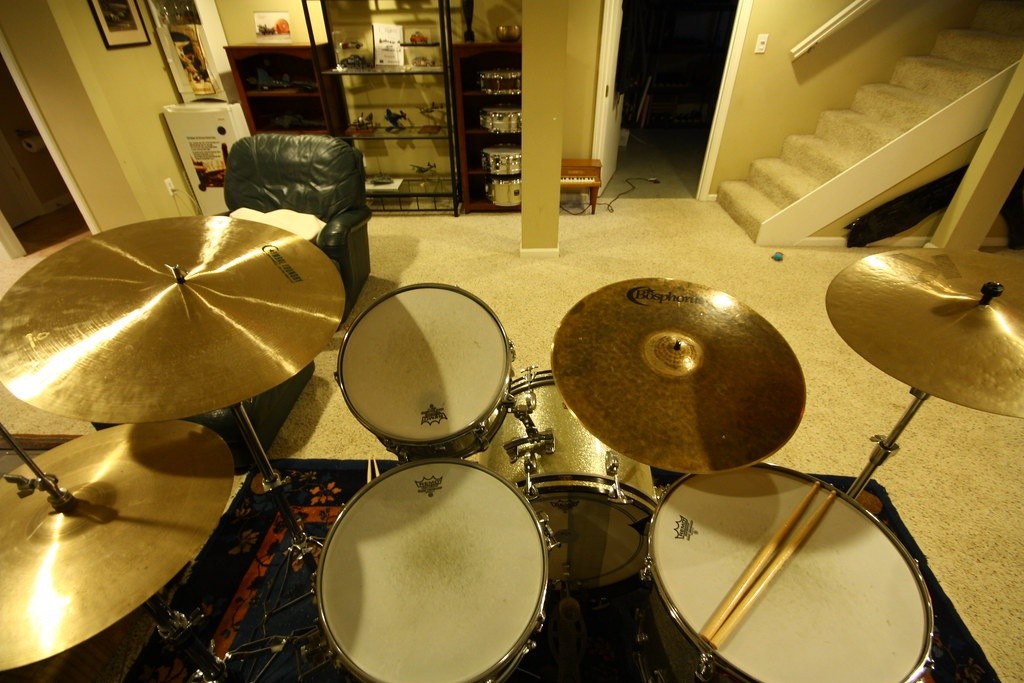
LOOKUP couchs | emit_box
[208,136,372,328]
[91,360,314,472]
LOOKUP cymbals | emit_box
[825,249,1024,419]
[0,215,346,424]
[552,277,803,473]
[0,423,231,671]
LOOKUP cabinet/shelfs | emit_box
[223,44,355,144]
[303,0,461,214]
[450,42,523,215]
[143,0,229,103]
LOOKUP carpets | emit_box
[121,458,1002,683]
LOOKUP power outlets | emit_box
[164,179,177,197]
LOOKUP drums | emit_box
[643,461,936,681]
[480,366,665,591]
[479,66,522,96]
[481,144,522,176]
[336,283,511,459]
[479,106,522,137]
[484,176,520,207]
[315,457,555,683]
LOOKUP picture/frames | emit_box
[86,0,152,50]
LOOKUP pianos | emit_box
[560,160,603,215]
